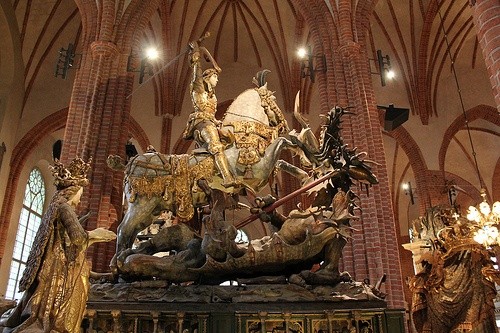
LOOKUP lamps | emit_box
[449,186,456,205]
[378,105,409,132]
[55,43,83,80]
[402,181,417,205]
[369,50,393,87]
[127,49,157,85]
[436,0,500,250]
[126,145,138,160]
[297,46,327,83]
[53,140,62,161]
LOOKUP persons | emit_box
[18,156,116,333]
[182,39,243,187]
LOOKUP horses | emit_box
[106,69,312,274]
